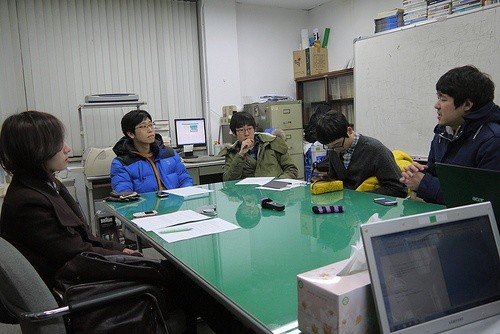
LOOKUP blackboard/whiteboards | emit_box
[353,3,500,163]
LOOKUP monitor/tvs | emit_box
[174,118,206,147]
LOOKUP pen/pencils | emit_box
[159,228,193,234]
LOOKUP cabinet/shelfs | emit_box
[296,68,354,127]
[252,100,304,181]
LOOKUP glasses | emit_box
[323,136,345,151]
[135,123,156,130]
[236,127,254,135]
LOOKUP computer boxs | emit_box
[180,148,208,157]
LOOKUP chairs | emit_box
[0,237,169,334]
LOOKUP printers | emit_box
[68,144,118,178]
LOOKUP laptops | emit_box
[360,200,500,334]
[435,162,500,233]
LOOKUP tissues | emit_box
[297,213,382,334]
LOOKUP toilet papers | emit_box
[301,30,309,49]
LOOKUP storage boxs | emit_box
[307,148,326,165]
[293,47,328,81]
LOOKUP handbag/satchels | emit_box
[50,251,174,333]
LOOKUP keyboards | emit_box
[184,156,225,163]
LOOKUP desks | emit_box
[84,156,225,239]
[103,178,447,334]
[77,102,147,154]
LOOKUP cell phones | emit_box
[132,210,158,218]
[374,198,398,206]
[156,192,169,197]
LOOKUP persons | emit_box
[316,111,408,198]
[217,112,299,179]
[399,64,500,207]
[0,111,202,334]
